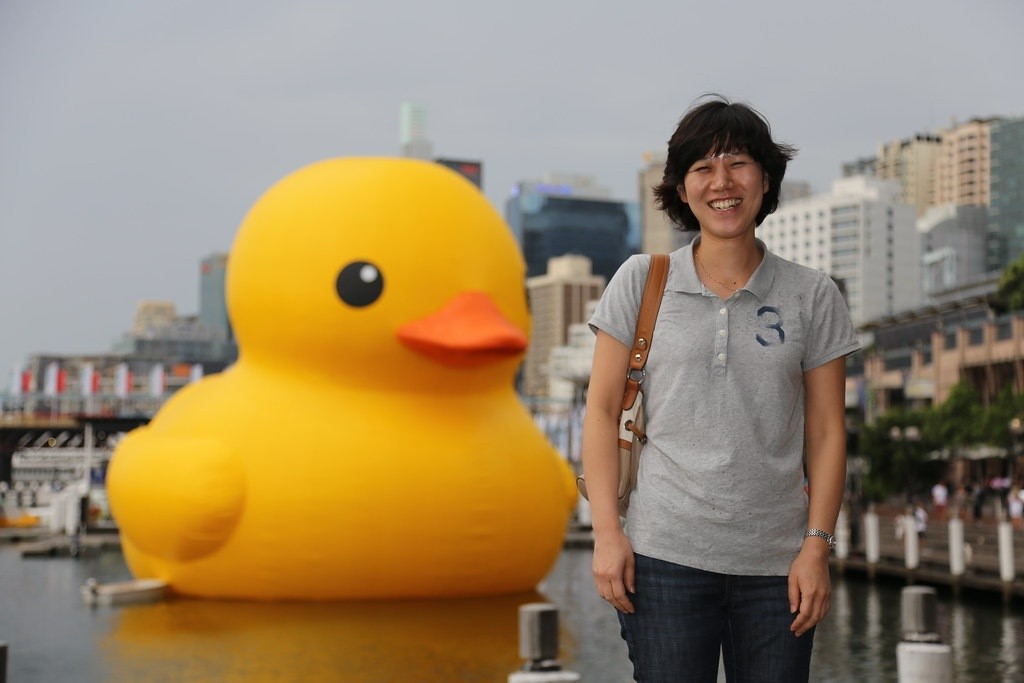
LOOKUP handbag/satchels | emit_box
[576,254,670,518]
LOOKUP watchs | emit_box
[804,528,836,550]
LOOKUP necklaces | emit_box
[695,250,737,293]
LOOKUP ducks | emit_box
[104,155,581,599]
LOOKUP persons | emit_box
[925,476,1024,531]
[583,92,863,682]
